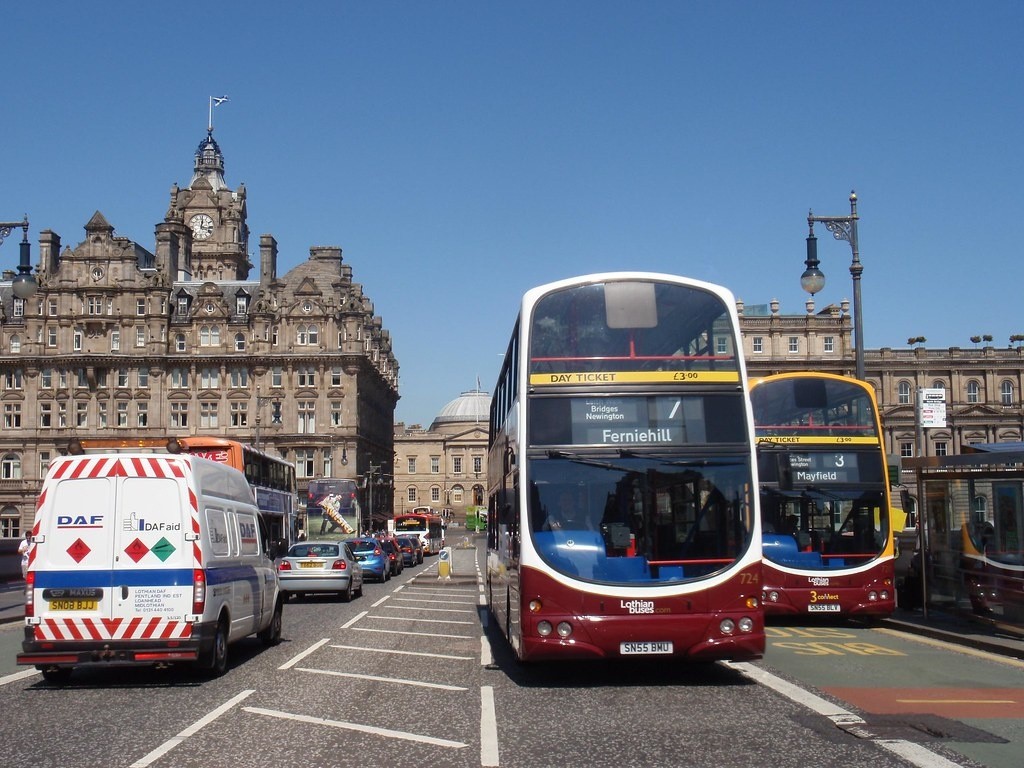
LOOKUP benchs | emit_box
[895,562,1023,638]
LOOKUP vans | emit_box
[16,454,283,683]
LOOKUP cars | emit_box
[407,536,426,564]
[393,537,417,567]
[343,538,391,583]
[277,541,364,604]
[371,537,405,577]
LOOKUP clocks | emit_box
[186,214,214,242]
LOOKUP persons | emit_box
[542,489,593,532]
[318,494,343,534]
[17,531,36,598]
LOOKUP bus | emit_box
[465,506,487,530]
[66,437,296,563]
[393,513,444,556]
[305,477,364,541]
[744,373,899,617]
[487,272,765,664]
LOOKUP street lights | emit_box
[369,459,387,533]
[800,189,863,381]
[254,384,281,451]
[330,434,347,478]
[444,488,452,509]
